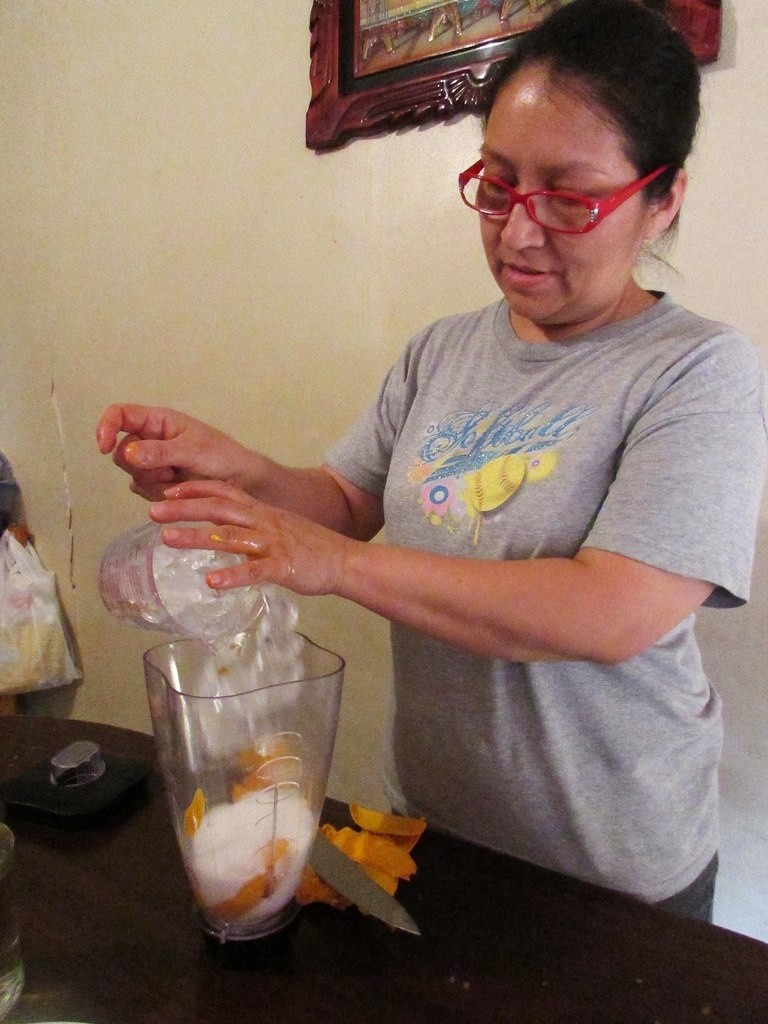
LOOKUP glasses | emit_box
[458,159,671,234]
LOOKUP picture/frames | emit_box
[306,0,722,148]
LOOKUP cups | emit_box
[0,821,25,1017]
[143,630,348,942]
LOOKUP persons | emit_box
[95,1,766,925]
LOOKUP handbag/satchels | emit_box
[0,528,84,695]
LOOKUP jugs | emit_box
[97,506,266,653]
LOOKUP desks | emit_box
[0,715,768,1024]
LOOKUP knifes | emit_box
[306,831,422,941]
